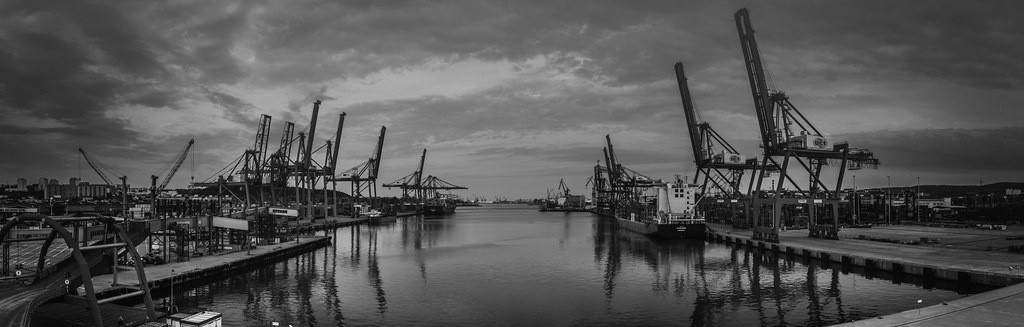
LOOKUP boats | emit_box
[603,133,707,241]
